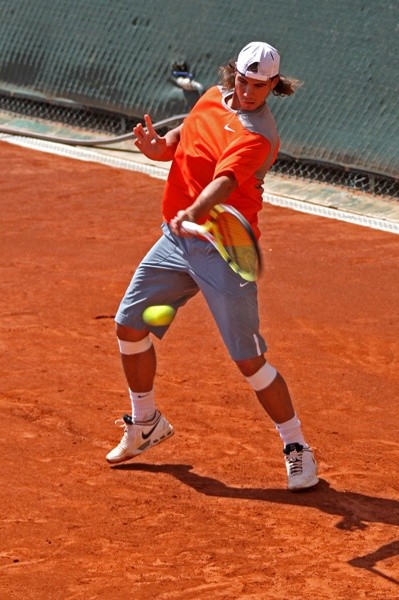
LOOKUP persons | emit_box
[105,40,320,494]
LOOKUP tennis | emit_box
[143,304,174,327]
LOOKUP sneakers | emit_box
[283,442,319,490]
[105,408,174,464]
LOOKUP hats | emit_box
[236,40,279,82]
